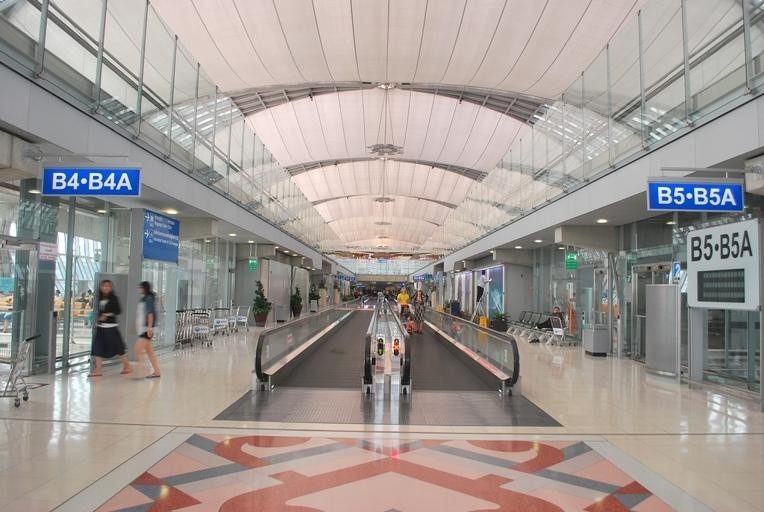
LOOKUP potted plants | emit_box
[288,283,303,318]
[491,304,511,332]
[249,278,274,326]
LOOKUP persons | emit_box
[54,289,95,328]
[87,280,134,376]
[0,291,13,333]
[412,290,425,334]
[475,271,491,302]
[395,287,409,317]
[528,307,564,343]
[126,280,161,380]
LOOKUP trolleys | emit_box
[177,305,254,349]
[1,333,40,408]
[547,316,578,347]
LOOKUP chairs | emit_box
[54,295,94,335]
[1,292,13,324]
[503,310,578,348]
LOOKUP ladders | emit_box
[470,297,486,322]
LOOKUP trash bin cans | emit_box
[451,303,459,315]
[583,323,608,357]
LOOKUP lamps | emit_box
[364,81,404,163]
[371,157,396,205]
[374,220,392,251]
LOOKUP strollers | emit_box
[398,304,416,336]
